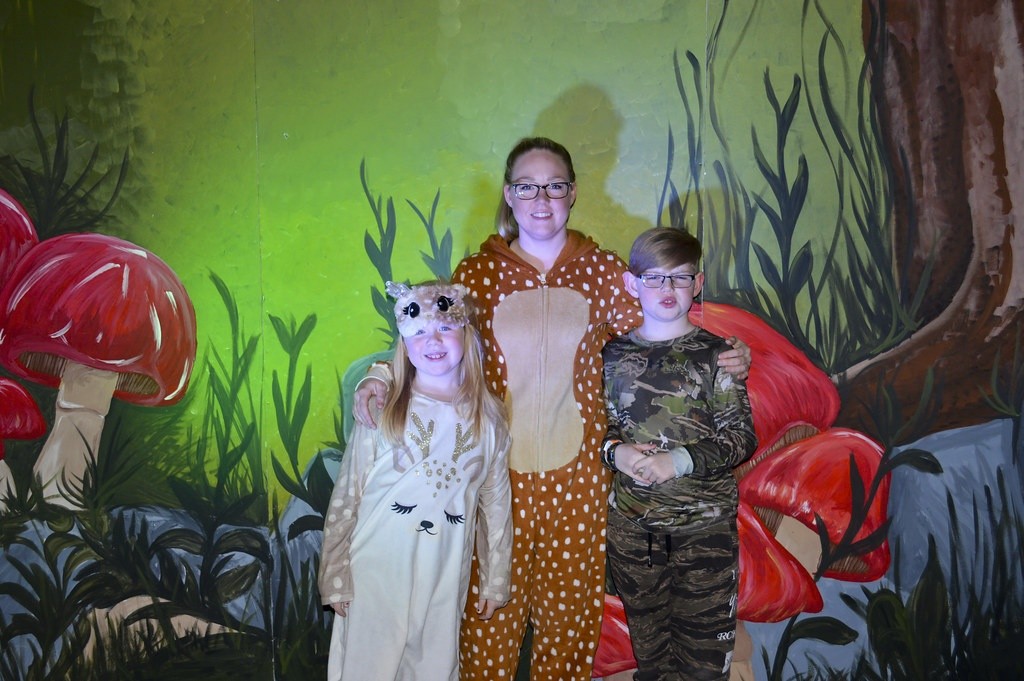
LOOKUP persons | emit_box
[318,135,757,681]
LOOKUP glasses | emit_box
[509,181,573,199]
[633,272,698,288]
